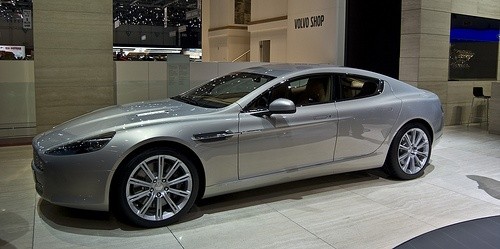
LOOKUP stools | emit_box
[467,87,492,131]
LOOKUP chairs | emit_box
[264,81,293,111]
[351,81,379,99]
[296,77,326,107]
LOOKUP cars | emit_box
[30,62,442,229]
